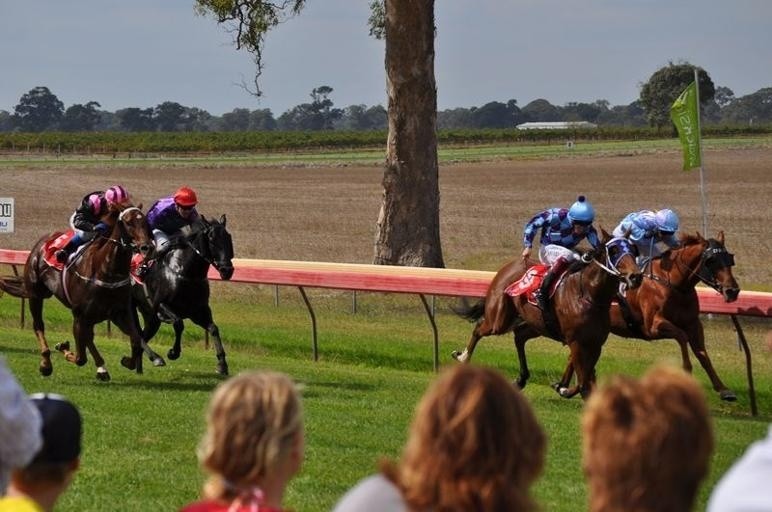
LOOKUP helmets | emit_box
[566,200,594,225]
[104,185,128,204]
[655,209,679,232]
[174,187,198,207]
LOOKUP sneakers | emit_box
[532,289,547,310]
[54,249,66,263]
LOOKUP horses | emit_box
[450,225,643,400]
[551,229,741,402]
[122,213,235,376]
[0,201,155,382]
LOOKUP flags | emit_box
[669,82,700,170]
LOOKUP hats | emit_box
[20,390,84,463]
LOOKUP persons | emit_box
[0,394,84,511]
[580,368,716,512]
[0,354,43,496]
[177,370,303,512]
[332,365,547,512]
[55,185,132,263]
[612,208,678,266]
[522,196,602,300]
[136,188,199,280]
